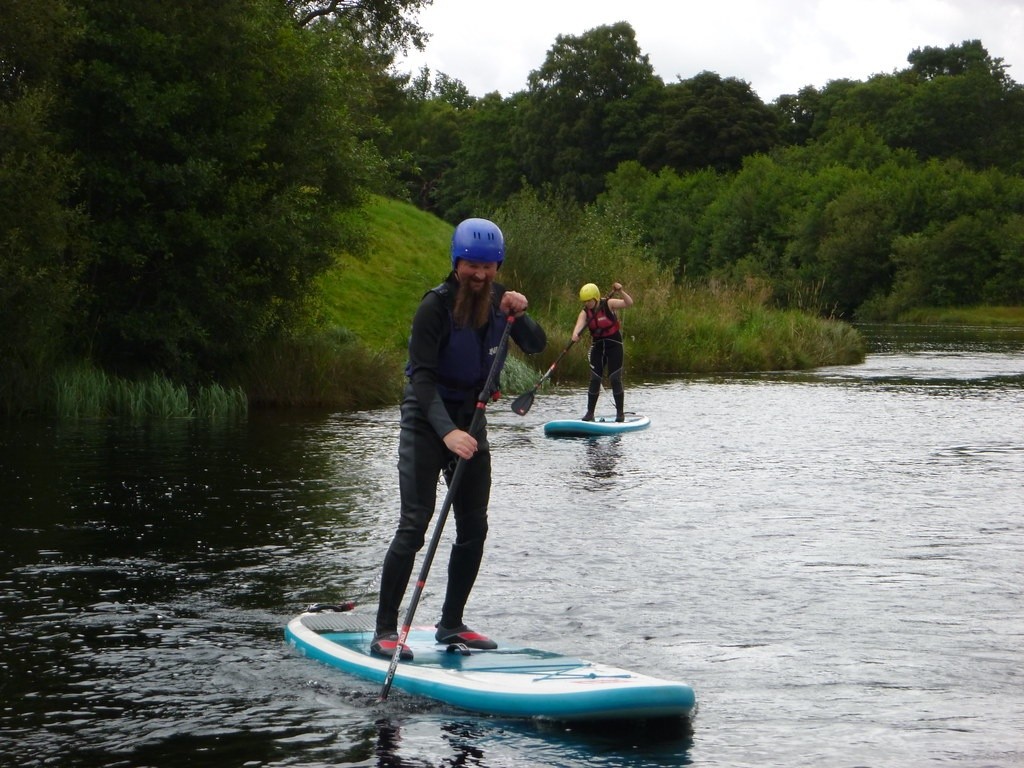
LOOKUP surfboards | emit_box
[544,411,651,436]
[287,601,695,723]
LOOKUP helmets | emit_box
[579,283,600,301]
[449,218,503,271]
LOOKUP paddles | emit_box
[381,307,519,706]
[510,289,616,416]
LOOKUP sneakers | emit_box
[434,621,497,649]
[370,632,414,661]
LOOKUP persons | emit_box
[570,280,632,423]
[371,216,548,659]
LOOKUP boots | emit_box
[581,391,599,421]
[613,391,625,422]
